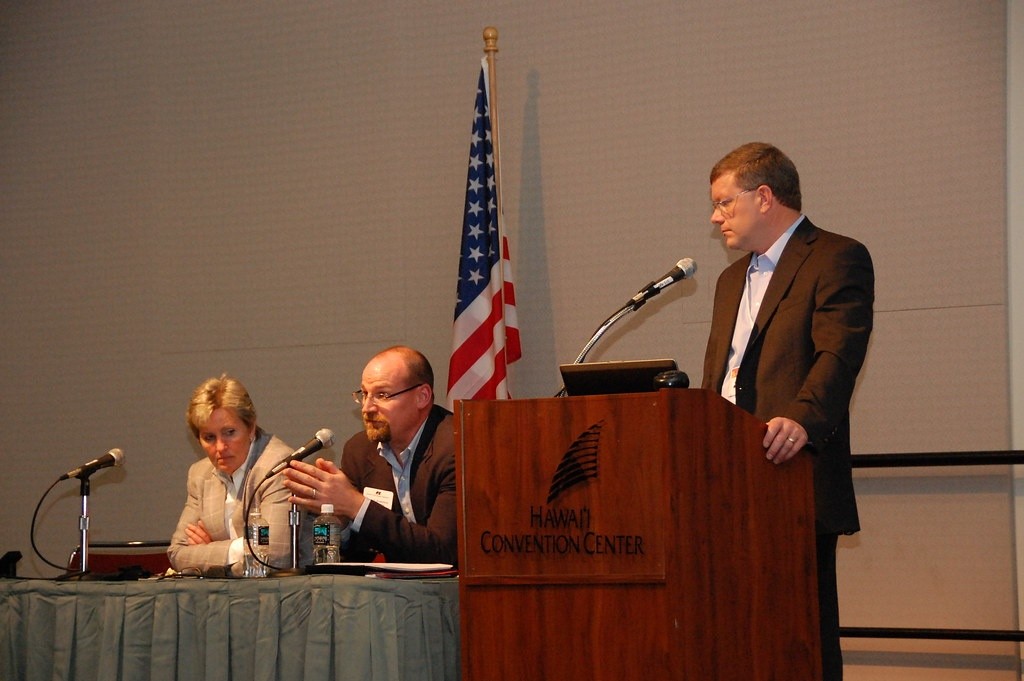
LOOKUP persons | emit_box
[701,142,875,681]
[283,345,458,577]
[167,373,302,577]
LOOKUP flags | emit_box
[446,55,522,414]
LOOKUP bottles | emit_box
[312,503,340,563]
[242,508,270,578]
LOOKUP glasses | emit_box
[710,186,760,213]
[351,384,424,405]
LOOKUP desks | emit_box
[0,573,460,680]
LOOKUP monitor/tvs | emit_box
[559,359,679,397]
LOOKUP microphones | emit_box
[60,448,126,481]
[264,428,336,480]
[628,257,697,306]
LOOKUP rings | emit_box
[313,488,316,499]
[787,437,796,444]
